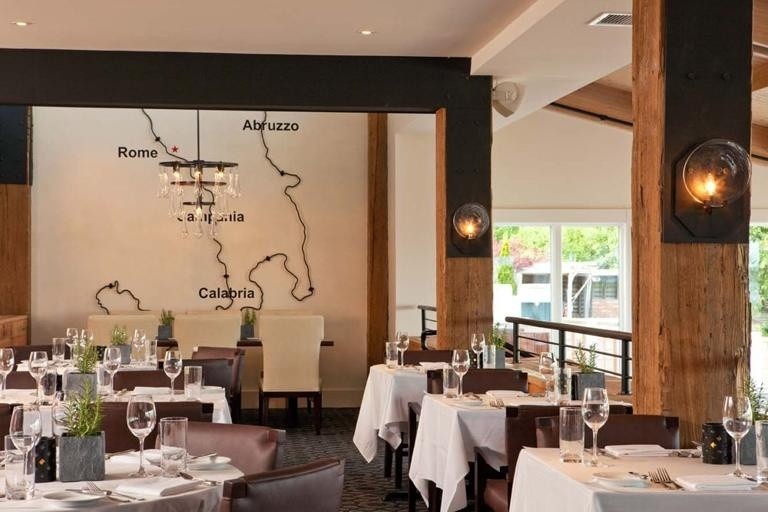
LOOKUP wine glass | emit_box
[581,387,609,468]
[395,332,557,402]
[0,328,183,499]
[721,395,754,480]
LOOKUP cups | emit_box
[386,341,573,408]
[753,420,768,485]
[558,407,584,464]
[4,337,202,500]
[702,423,732,464]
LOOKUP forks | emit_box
[657,467,686,492]
[648,470,674,491]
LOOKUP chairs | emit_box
[463,368,528,395]
[484,405,631,511]
[398,350,452,365]
[534,413,663,447]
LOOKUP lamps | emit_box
[450,202,491,255]
[492,80,526,119]
[156,109,240,239]
[675,138,753,238]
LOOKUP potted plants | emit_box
[730,380,768,465]
[484,321,508,370]
[570,344,605,400]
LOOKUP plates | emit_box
[187,456,232,471]
[419,361,526,405]
[592,471,650,487]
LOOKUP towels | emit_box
[419,361,449,369]
[604,444,669,458]
[486,390,527,397]
[676,475,756,491]
